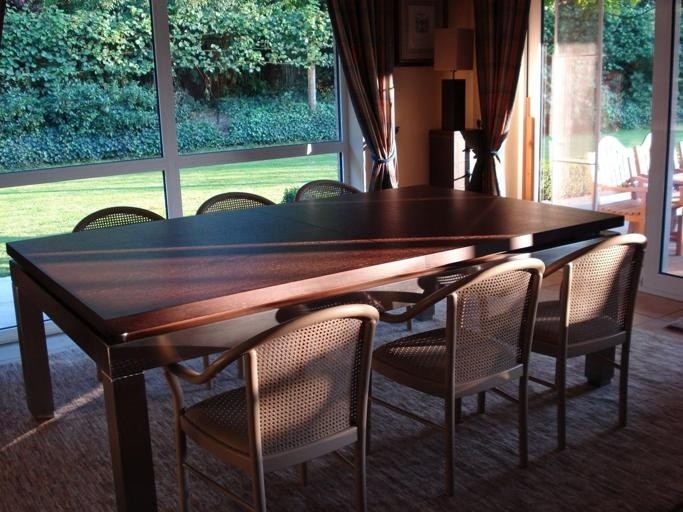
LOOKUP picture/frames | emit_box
[391,0,449,67]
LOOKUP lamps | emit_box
[431,29,475,130]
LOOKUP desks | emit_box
[2,182,623,511]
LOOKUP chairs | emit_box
[365,259,545,497]
[585,133,683,256]
[75,176,416,389]
[477,233,645,451]
[164,303,379,507]
